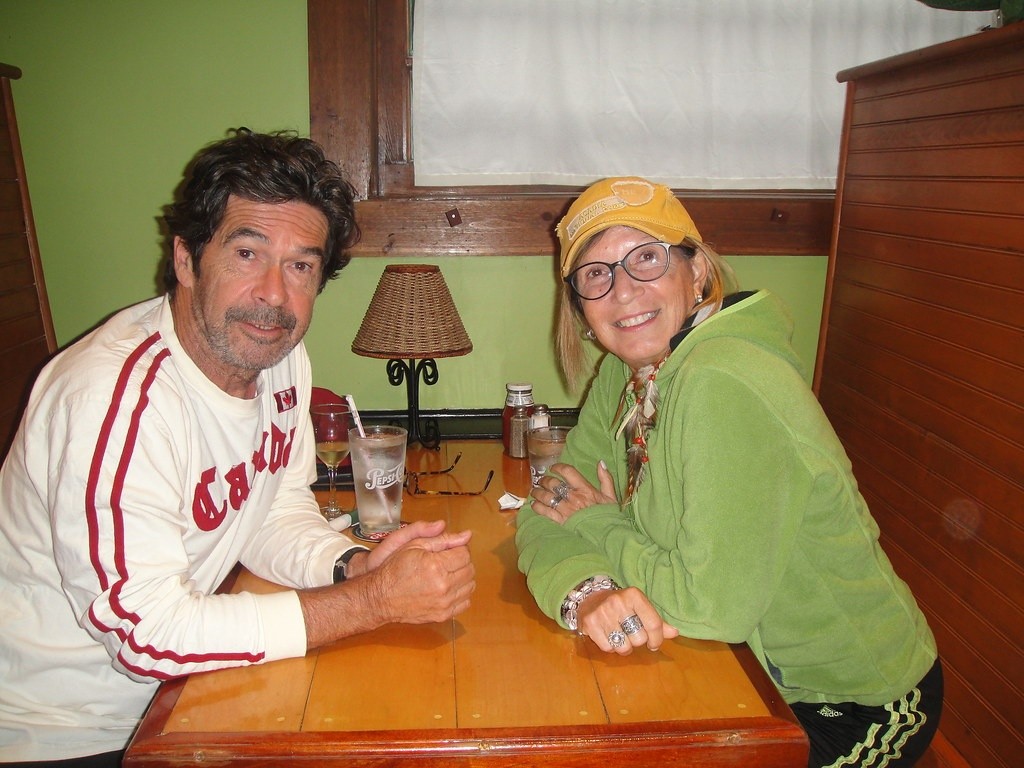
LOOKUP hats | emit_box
[555,176,702,284]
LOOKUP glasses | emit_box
[403,452,494,496]
[563,241,671,300]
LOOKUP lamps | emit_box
[351,264,474,451]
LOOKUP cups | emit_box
[347,426,407,538]
[527,426,574,488]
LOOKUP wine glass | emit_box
[309,404,352,520]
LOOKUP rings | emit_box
[550,495,562,509]
[620,613,643,635]
[609,631,626,647]
[553,484,569,499]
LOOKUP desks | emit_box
[123,437,811,768]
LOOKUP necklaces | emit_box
[609,350,669,500]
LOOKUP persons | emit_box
[514,179,941,767]
[0,127,477,768]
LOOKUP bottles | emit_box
[502,383,536,451]
[527,404,551,434]
[509,406,532,459]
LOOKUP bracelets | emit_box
[561,575,622,631]
[333,547,370,584]
